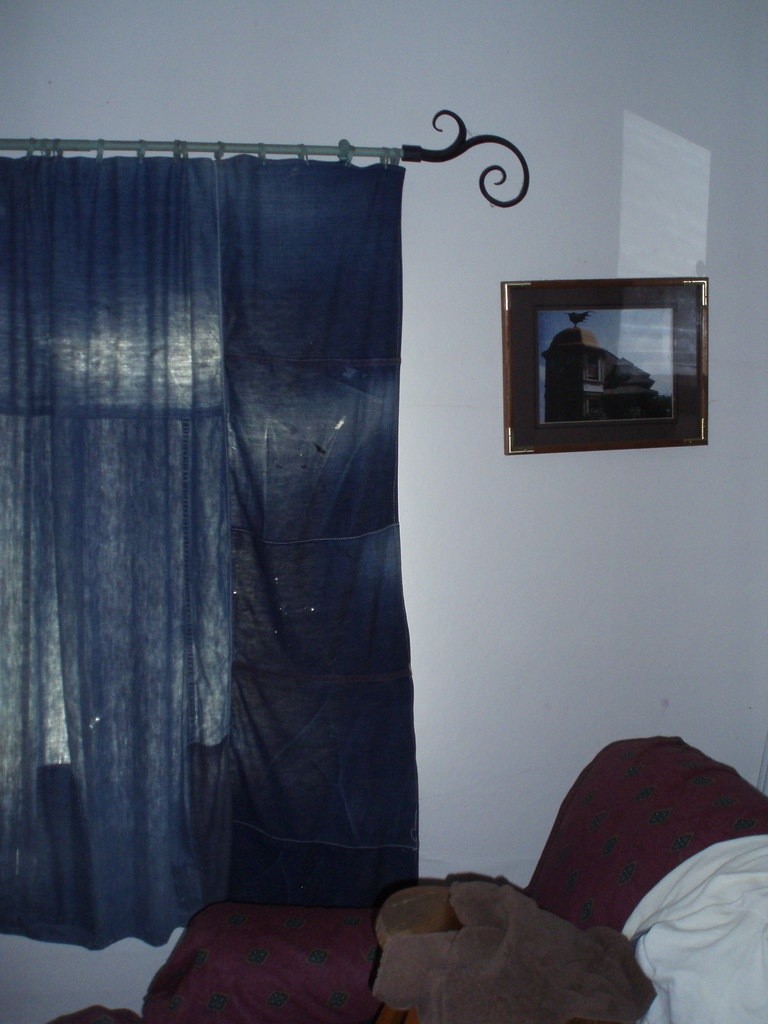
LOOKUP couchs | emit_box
[141,736,768,1024]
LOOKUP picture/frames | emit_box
[499,277,707,450]
